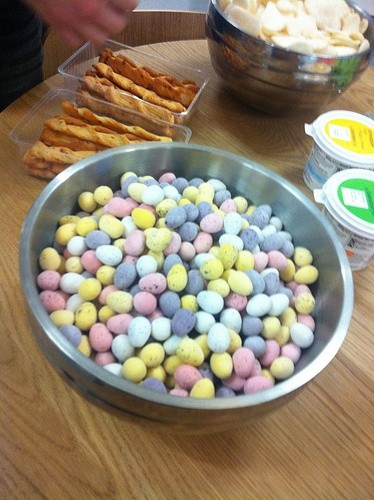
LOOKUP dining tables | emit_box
[0,40,374,500]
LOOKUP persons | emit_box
[1,0,142,109]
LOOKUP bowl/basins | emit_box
[20,141,355,434]
[206,1,374,115]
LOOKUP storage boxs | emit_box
[10,88,192,181]
[56,38,211,126]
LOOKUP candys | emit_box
[35,167,322,403]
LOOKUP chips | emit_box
[214,0,372,56]
